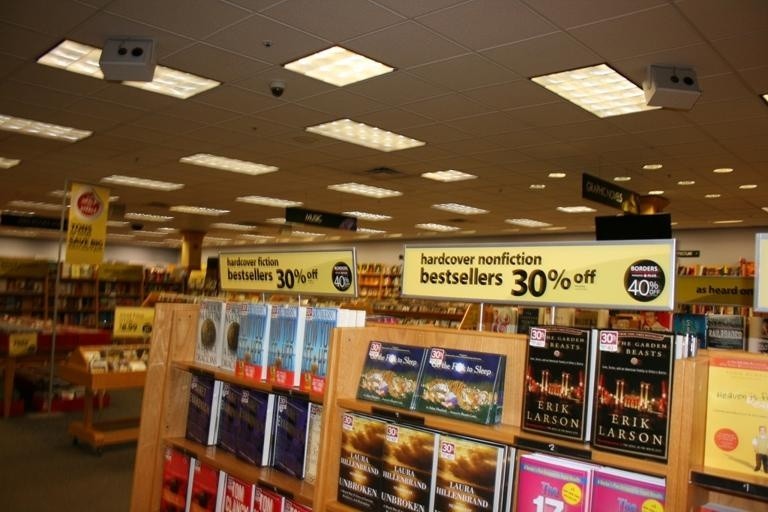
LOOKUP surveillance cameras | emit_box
[270,62,291,97]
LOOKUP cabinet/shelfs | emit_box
[0,260,145,326]
[676,266,755,314]
[344,266,496,331]
[66,343,150,451]
[130,303,336,512]
[332,329,768,511]
[2,328,110,413]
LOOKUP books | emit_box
[99,279,140,294]
[434,434,516,512]
[338,412,394,512]
[589,467,666,512]
[1,294,43,307]
[416,348,507,425]
[96,315,113,329]
[378,427,440,512]
[147,269,182,282]
[677,257,757,278]
[193,300,367,398]
[676,303,751,352]
[144,284,182,294]
[56,312,96,327]
[356,261,405,298]
[703,359,767,479]
[591,330,697,464]
[75,344,153,377]
[483,305,674,334]
[520,327,596,443]
[98,297,139,312]
[156,450,312,511]
[1,276,44,292]
[0,311,57,336]
[371,297,474,329]
[516,453,597,512]
[185,373,322,483]
[52,282,94,295]
[356,342,426,409]
[48,295,94,310]
